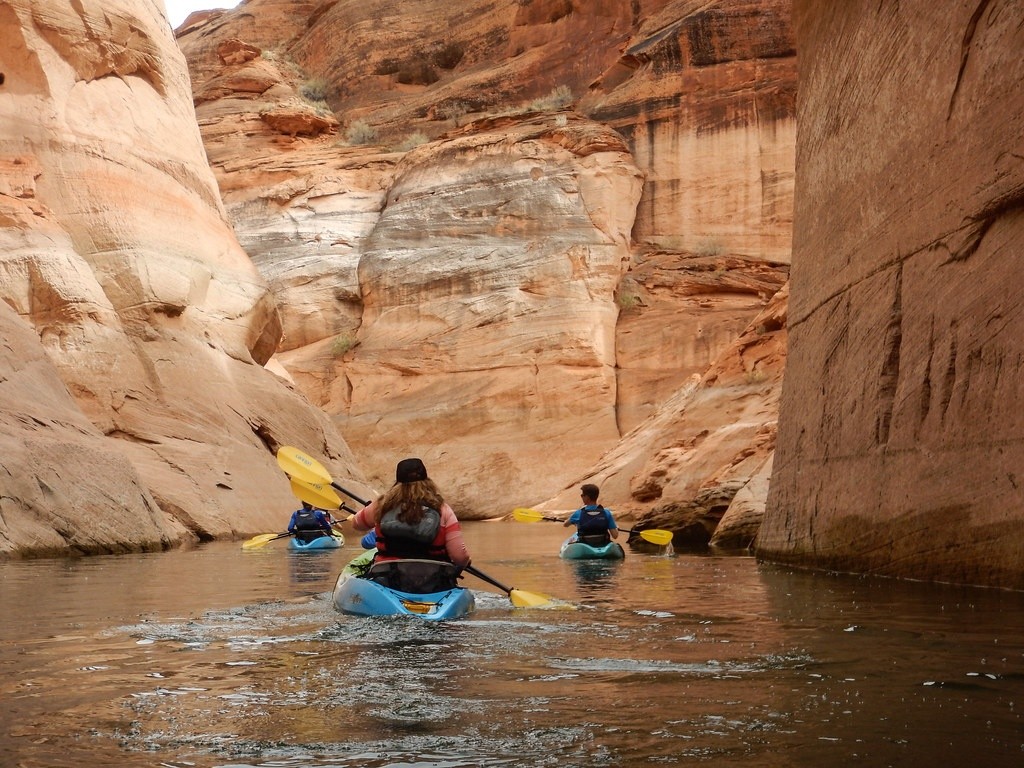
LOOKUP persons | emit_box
[314,507,343,530]
[352,458,471,593]
[564,484,618,547]
[288,500,332,541]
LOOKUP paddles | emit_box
[276,445,570,612]
[242,513,355,551]
[513,507,674,546]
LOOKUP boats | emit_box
[287,528,344,552]
[333,544,475,621]
[559,531,625,560]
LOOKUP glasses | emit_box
[581,494,585,497]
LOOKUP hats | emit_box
[395,457,428,484]
